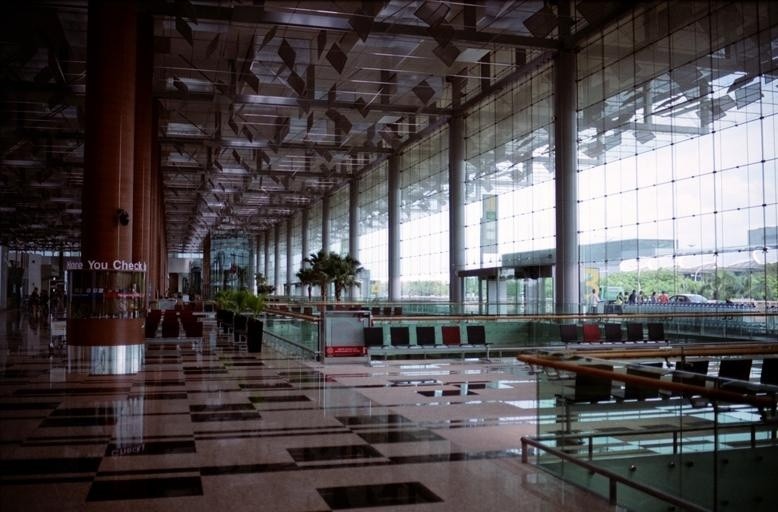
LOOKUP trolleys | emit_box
[47,279,67,354]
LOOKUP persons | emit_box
[622,291,628,304]
[627,289,636,304]
[616,292,622,312]
[721,298,734,320]
[591,289,599,312]
[26,286,50,328]
[650,292,657,304]
[636,291,643,305]
[598,287,604,301]
[656,291,668,303]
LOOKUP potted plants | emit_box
[212,288,271,353]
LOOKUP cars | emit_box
[668,294,709,304]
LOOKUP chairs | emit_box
[144,307,203,339]
[264,297,493,364]
[558,321,670,345]
[553,356,776,439]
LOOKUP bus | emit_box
[597,285,623,301]
